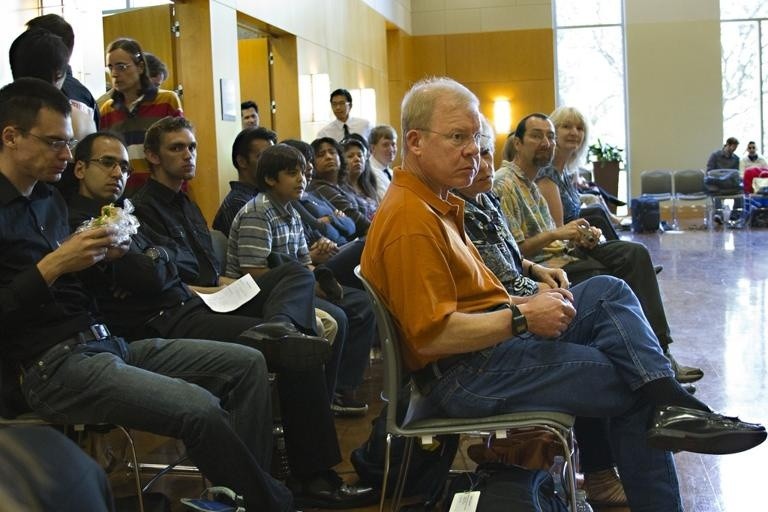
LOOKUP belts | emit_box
[10,323,112,379]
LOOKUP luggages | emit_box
[631,196,659,234]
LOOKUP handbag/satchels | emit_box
[350,403,441,496]
[180,486,245,512]
[703,168,742,196]
[744,167,768,192]
[441,462,572,512]
[752,171,768,194]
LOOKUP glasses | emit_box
[330,100,351,107]
[105,60,134,70]
[15,128,79,152]
[86,156,134,174]
[411,127,481,145]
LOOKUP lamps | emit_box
[494,99,510,133]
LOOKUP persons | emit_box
[68,134,379,512]
[0,77,351,512]
[360,74,767,512]
[452,110,630,511]
[706,136,768,229]
[0,15,708,411]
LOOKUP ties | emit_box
[384,169,392,181]
[343,124,349,135]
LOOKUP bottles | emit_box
[567,489,594,512]
[549,456,566,499]
[272,416,289,472]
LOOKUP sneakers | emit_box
[667,353,703,382]
[713,213,724,224]
[330,392,369,416]
[582,468,630,504]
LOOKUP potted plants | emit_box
[585,140,624,218]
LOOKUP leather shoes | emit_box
[680,383,696,394]
[239,322,332,367]
[285,469,375,509]
[654,264,664,274]
[649,404,767,454]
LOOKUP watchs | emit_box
[506,304,527,336]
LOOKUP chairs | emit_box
[706,169,748,231]
[674,169,715,232]
[352,264,578,512]
[0,394,204,510]
[640,170,673,232]
